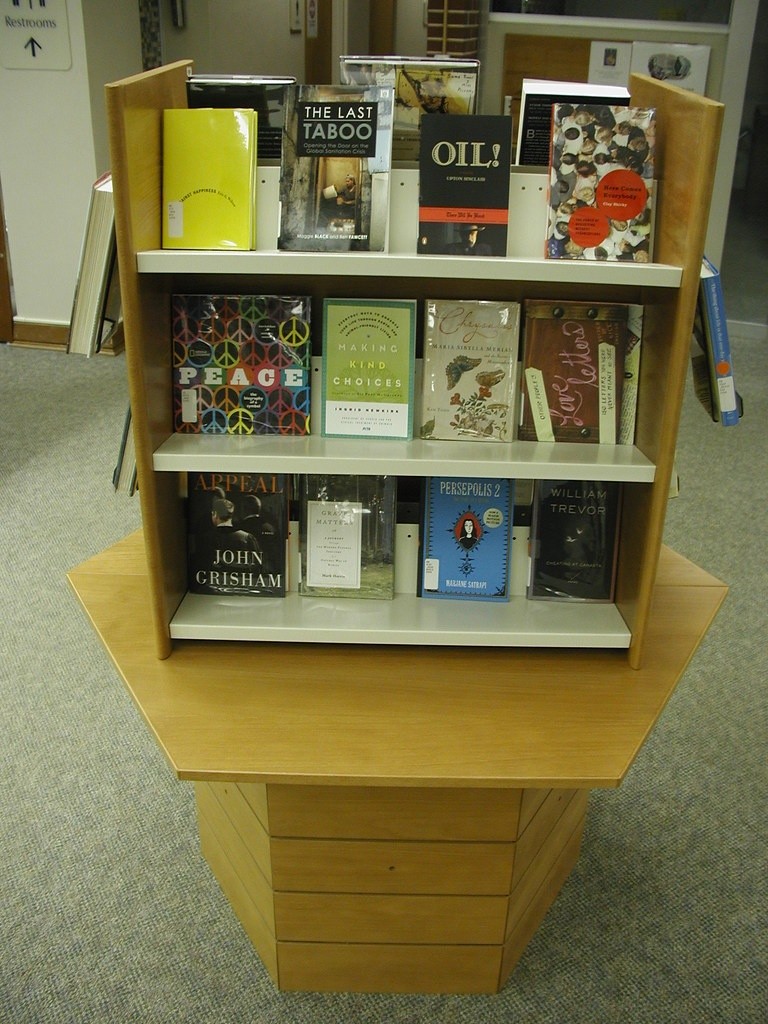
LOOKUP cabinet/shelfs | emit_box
[55,60,730,999]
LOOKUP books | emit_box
[691,255,739,428]
[65,170,120,360]
[113,399,137,499]
[163,292,644,447]
[159,54,659,266]
[185,472,625,603]
